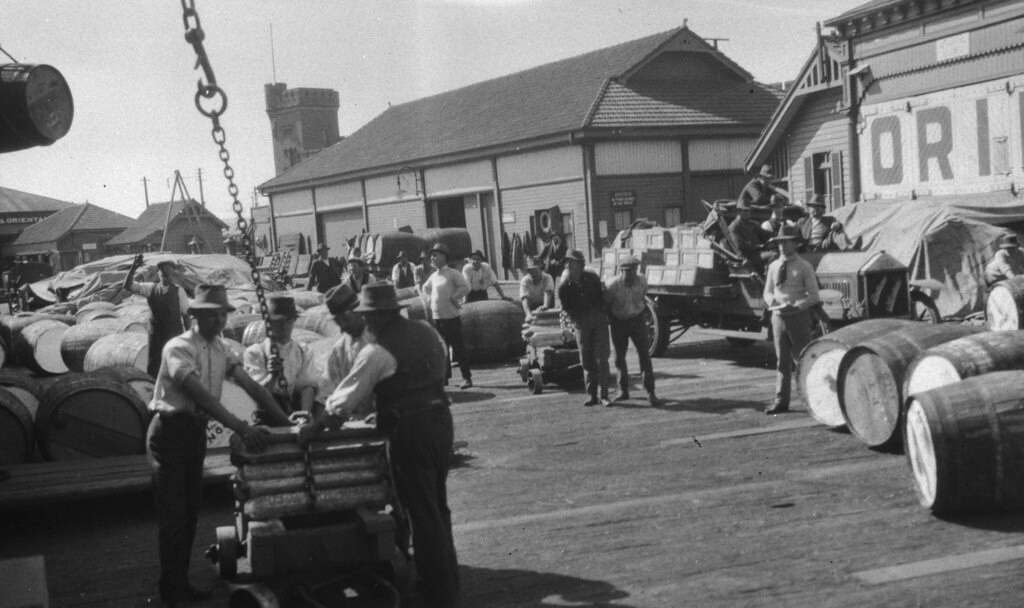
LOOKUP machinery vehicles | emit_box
[604,198,941,358]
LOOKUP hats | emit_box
[999,234,1019,248]
[757,164,775,179]
[188,285,236,315]
[353,282,409,312]
[525,255,545,271]
[324,283,361,320]
[419,250,429,261]
[732,199,754,211]
[560,249,585,266]
[768,194,786,207]
[469,250,484,262]
[550,229,562,239]
[427,243,450,262]
[782,204,803,218]
[348,255,365,268]
[395,251,409,259]
[617,256,640,270]
[316,244,330,252]
[267,296,302,321]
[769,225,799,241]
[806,193,825,208]
[157,260,176,269]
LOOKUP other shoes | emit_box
[600,397,611,407]
[460,379,472,389]
[166,584,212,608]
[614,392,629,400]
[402,576,438,607]
[848,236,863,252]
[765,404,788,413]
[648,393,660,405]
[584,395,597,406]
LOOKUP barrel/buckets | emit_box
[794,272,1024,521]
[0,226,529,607]
[0,62,74,154]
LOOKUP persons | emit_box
[123,255,191,382]
[460,250,514,301]
[981,235,1024,321]
[422,243,474,389]
[146,282,312,608]
[540,234,570,283]
[307,242,342,294]
[343,248,437,297]
[313,284,384,425]
[519,256,553,323]
[297,282,460,608]
[728,163,863,278]
[558,249,612,406]
[603,257,663,406]
[244,293,320,424]
[763,224,820,415]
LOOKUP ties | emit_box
[776,259,786,286]
